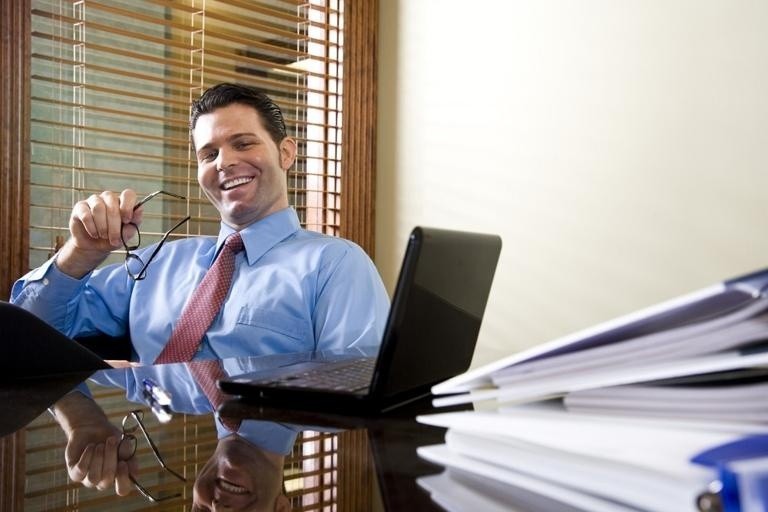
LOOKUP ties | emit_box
[148,228,245,366]
[182,361,243,431]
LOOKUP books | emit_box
[414,270,767,511]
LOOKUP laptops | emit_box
[218,399,447,510]
[215,227,502,413]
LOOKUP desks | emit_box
[0,344,472,512]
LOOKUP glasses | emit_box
[120,185,190,284]
[115,408,188,505]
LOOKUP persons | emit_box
[45,365,350,512]
[7,82,393,365]
